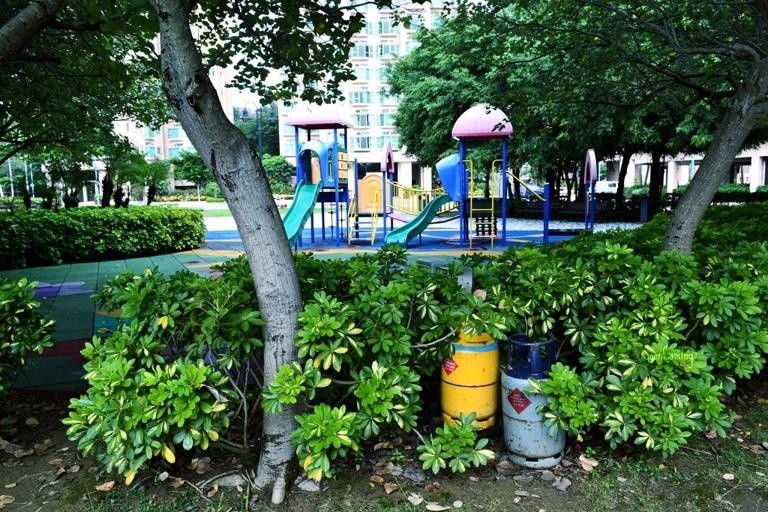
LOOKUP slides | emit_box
[280,179,323,243]
[384,193,453,252]
